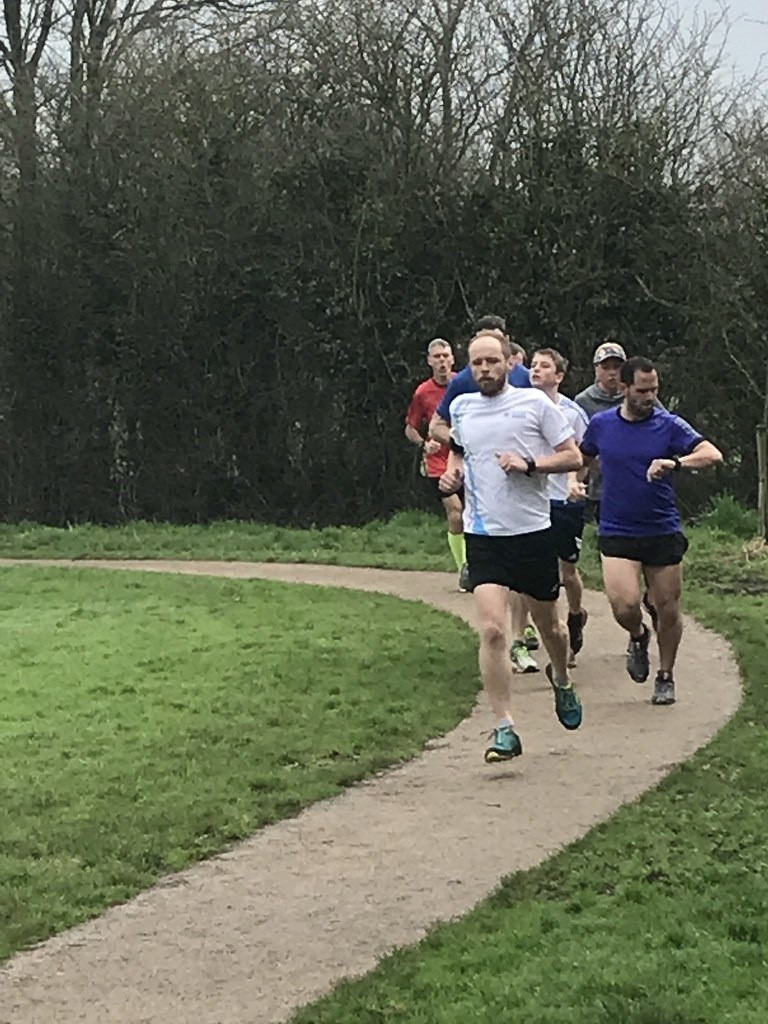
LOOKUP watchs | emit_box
[671,456,681,471]
[419,440,425,448]
[523,457,535,478]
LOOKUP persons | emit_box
[438,329,584,763]
[574,342,664,631]
[428,315,539,674]
[405,340,469,593]
[565,356,724,705]
[530,347,589,654]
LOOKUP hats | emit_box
[594,342,627,365]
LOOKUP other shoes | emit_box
[459,563,470,593]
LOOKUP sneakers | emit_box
[480,726,521,763]
[626,622,651,682]
[566,607,587,654]
[545,663,581,730]
[642,592,658,632]
[651,670,676,705]
[523,625,539,649]
[509,639,540,673]
